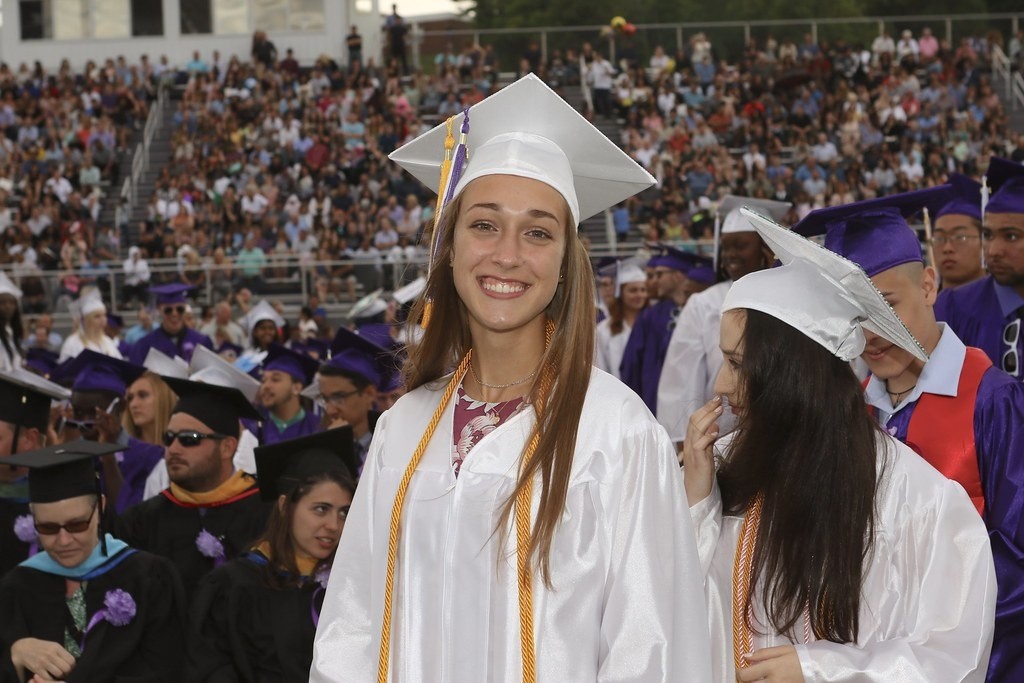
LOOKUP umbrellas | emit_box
[771,74,816,94]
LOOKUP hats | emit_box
[261,342,319,387]
[647,244,716,284]
[240,300,286,343]
[67,286,105,333]
[253,425,358,505]
[188,344,259,404]
[1,439,129,556]
[389,71,657,329]
[324,321,408,391]
[160,377,261,437]
[984,156,1024,213]
[935,175,983,220]
[0,270,23,304]
[147,280,196,304]
[0,368,72,471]
[598,255,648,298]
[790,185,951,276]
[73,349,147,401]
[719,206,932,366]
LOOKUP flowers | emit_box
[313,564,331,590]
[98,588,138,628]
[194,528,226,560]
[183,342,195,351]
[13,514,41,543]
[115,452,124,464]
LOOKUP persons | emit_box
[0,4,1024,311]
[935,153,1024,381]
[0,270,461,683]
[309,67,710,683]
[592,195,792,457]
[679,206,998,683]
[915,172,990,289]
[791,181,1024,683]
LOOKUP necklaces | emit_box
[885,386,915,408]
[467,358,541,415]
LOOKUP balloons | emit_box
[600,26,616,38]
[611,16,626,32]
[621,23,636,37]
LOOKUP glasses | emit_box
[162,429,222,447]
[33,501,98,535]
[315,389,360,405]
[62,397,119,432]
[929,233,980,247]
[160,305,185,314]
